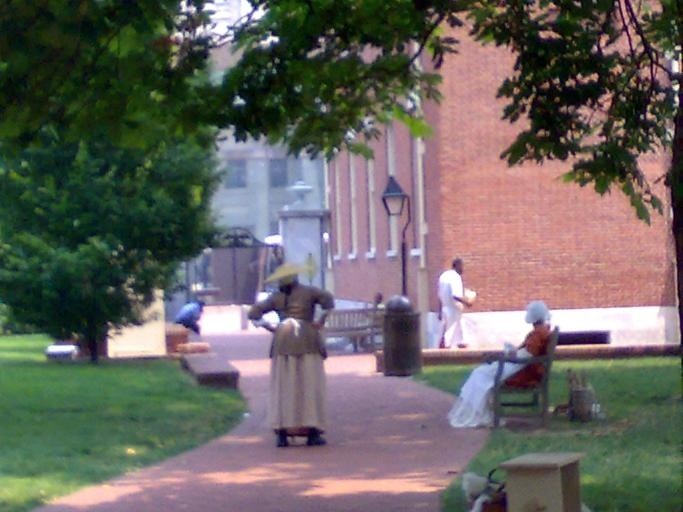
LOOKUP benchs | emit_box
[486,327,559,428]
[317,302,386,357]
[554,327,610,346]
[499,449,585,511]
[177,339,210,357]
[164,320,188,352]
[182,348,241,388]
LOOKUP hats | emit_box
[261,263,320,283]
[524,298,551,324]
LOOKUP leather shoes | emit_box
[307,435,327,444]
[276,439,289,446]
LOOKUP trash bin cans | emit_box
[384,296,422,376]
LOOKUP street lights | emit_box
[380,171,413,298]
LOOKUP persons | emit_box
[437,258,473,348]
[175,300,205,334]
[248,263,335,446]
[446,300,551,427]
